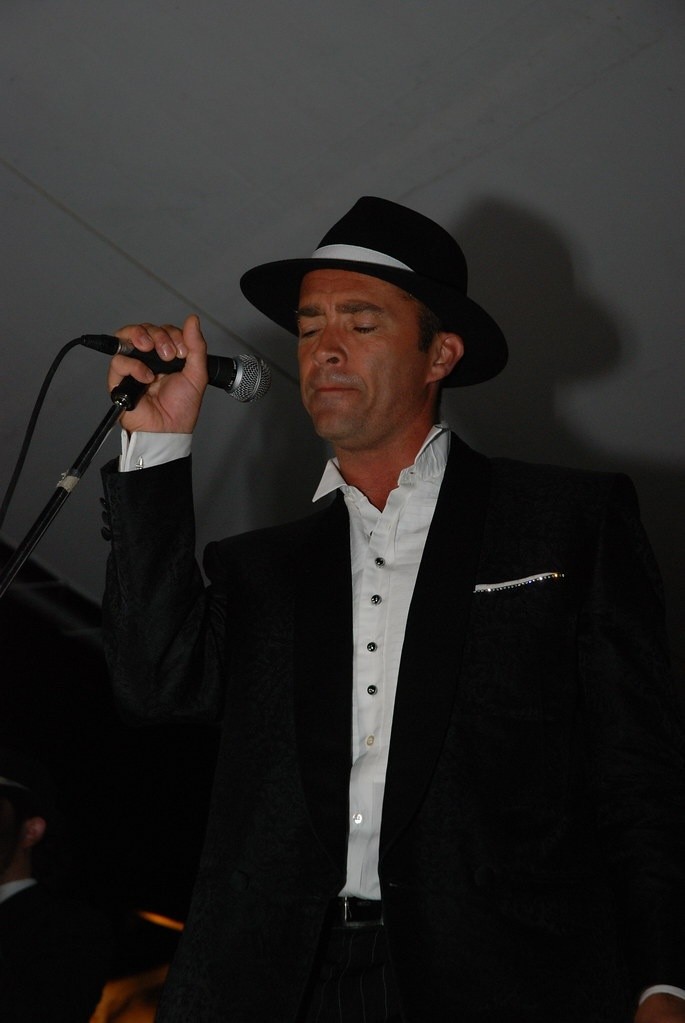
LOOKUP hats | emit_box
[0,748,65,843]
[240,196,508,389]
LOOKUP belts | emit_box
[323,898,383,929]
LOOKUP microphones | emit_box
[80,333,273,403]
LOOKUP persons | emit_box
[1,741,81,1022]
[88,199,685,1023]
